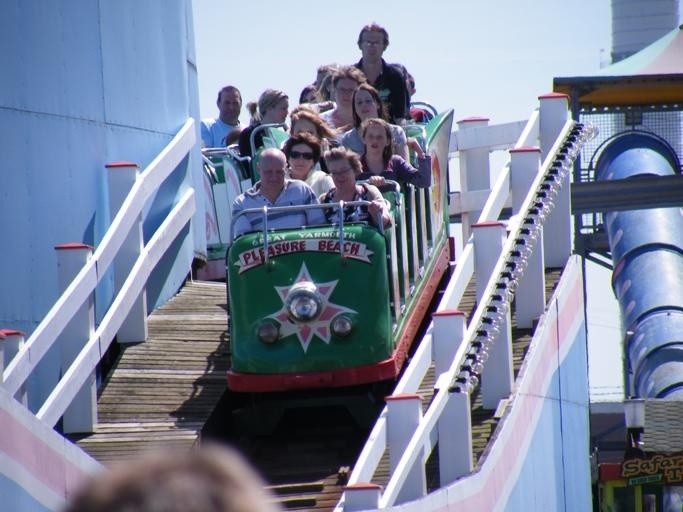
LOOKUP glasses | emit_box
[328,167,353,177]
[288,150,315,160]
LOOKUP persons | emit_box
[285,21,434,181]
[197,84,246,150]
[229,147,327,241]
[237,88,291,170]
[280,133,334,196]
[315,147,392,236]
[350,118,432,192]
